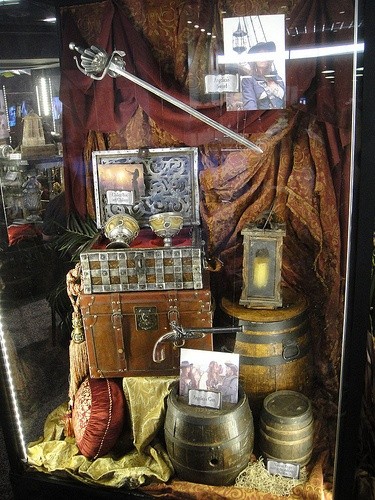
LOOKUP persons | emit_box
[125,168,140,201]
[181,360,238,403]
[241,41,286,110]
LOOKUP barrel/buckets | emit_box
[164,381,254,487]
[257,390,316,469]
[214,290,315,413]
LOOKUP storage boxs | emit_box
[81,270,212,380]
[75,146,203,294]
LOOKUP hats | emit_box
[247,42,275,65]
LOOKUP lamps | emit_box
[233,18,247,55]
[238,208,286,308]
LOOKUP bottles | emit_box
[21,109,46,146]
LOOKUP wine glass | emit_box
[103,213,139,249]
[148,211,184,248]
